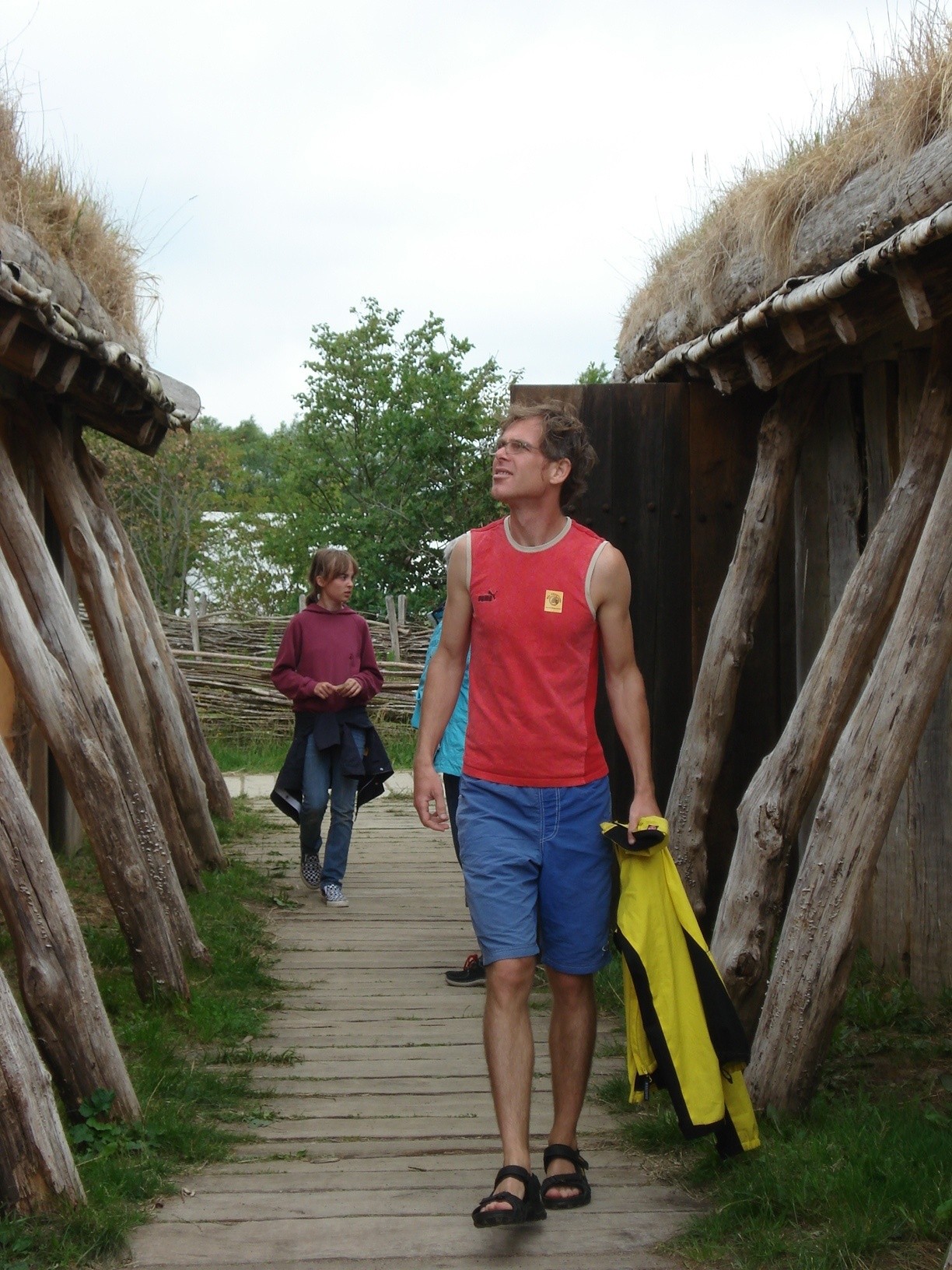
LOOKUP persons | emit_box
[270,548,385,907]
[413,535,488,987]
[413,403,664,1229]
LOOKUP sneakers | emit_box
[300,850,322,888]
[444,955,486,986]
[321,883,349,907]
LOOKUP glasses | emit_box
[496,439,560,462]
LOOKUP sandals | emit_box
[541,1144,590,1208]
[473,1165,546,1226]
